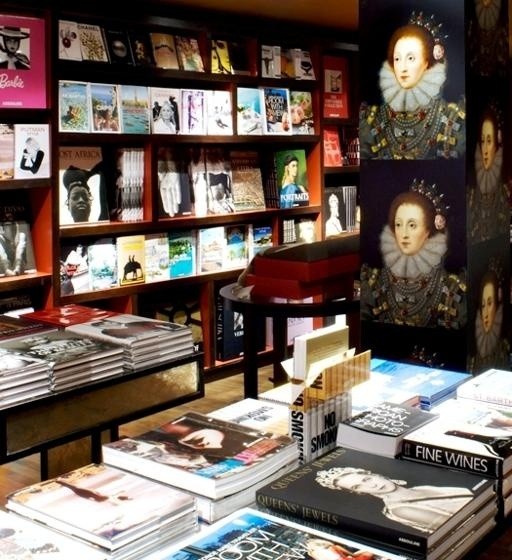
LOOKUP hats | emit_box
[0,26,29,38]
[63,165,91,194]
[285,155,298,165]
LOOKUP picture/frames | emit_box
[58,80,293,136]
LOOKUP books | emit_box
[1,0,512,559]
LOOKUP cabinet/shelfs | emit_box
[0,0,359,382]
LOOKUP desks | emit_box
[0,353,206,482]
[219,281,361,399]
